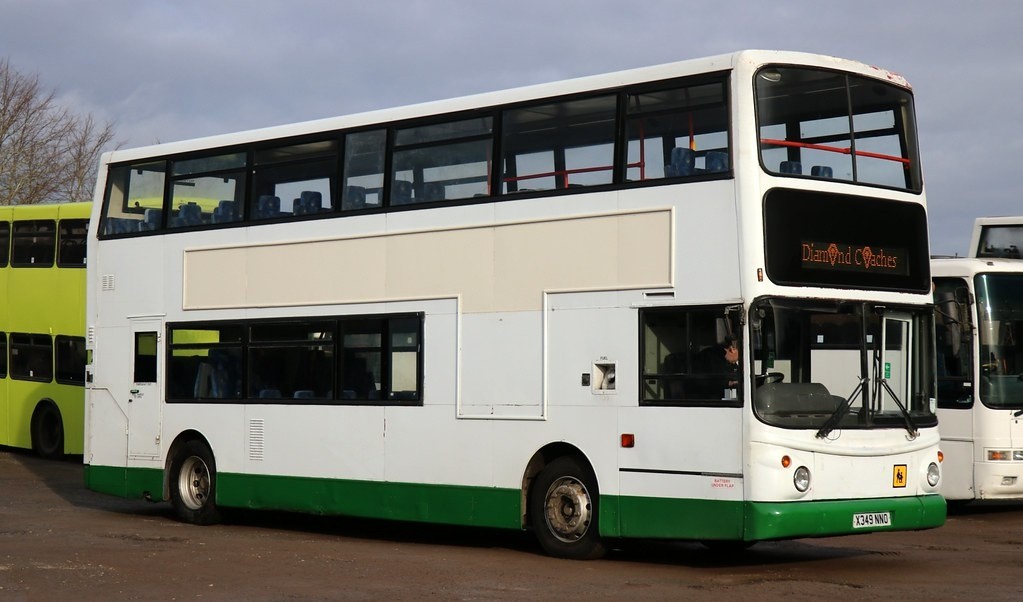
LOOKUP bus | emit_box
[0,195,221,460]
[76,47,974,559]
[724,214,1023,513]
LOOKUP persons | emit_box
[692,335,738,400]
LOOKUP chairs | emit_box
[14,239,87,266]
[664,147,728,177]
[107,180,445,237]
[811,166,832,177]
[663,352,689,400]
[780,162,802,174]
[259,389,416,399]
